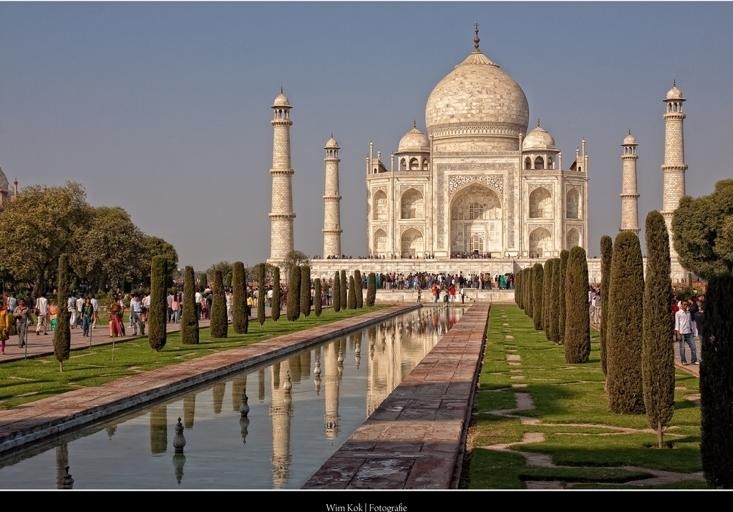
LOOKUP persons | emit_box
[357,266,517,303]
[224,286,290,322]
[587,284,705,369]
[0,291,99,347]
[309,286,332,306]
[109,286,215,337]
[312,254,434,259]
[449,251,491,259]
